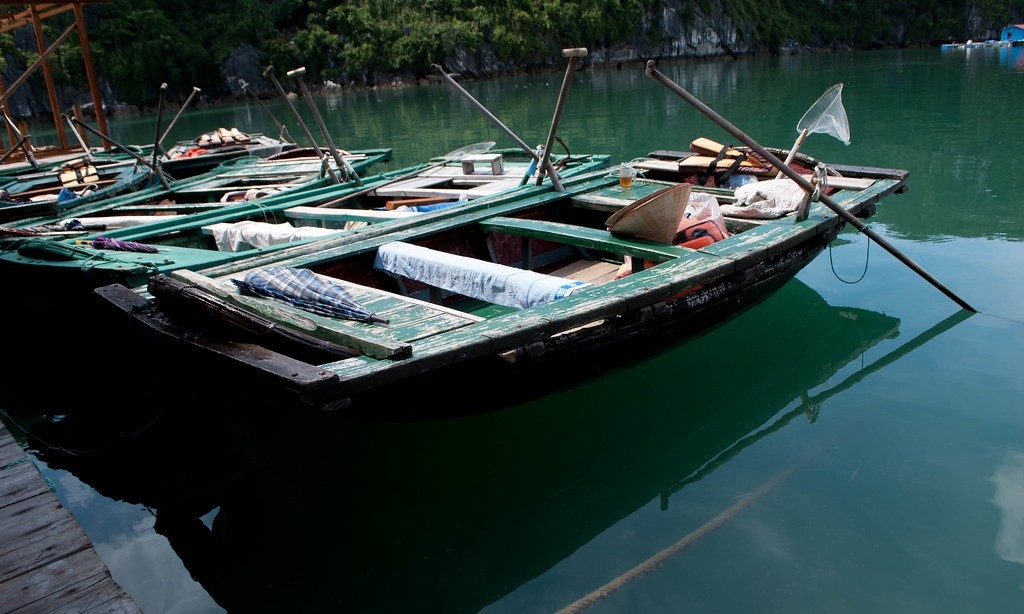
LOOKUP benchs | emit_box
[197,223,341,243]
[174,185,294,195]
[480,216,697,275]
[372,241,592,305]
[214,269,486,343]
[113,202,231,211]
[283,206,425,228]
[56,214,184,228]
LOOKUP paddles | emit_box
[0,131,32,164]
[531,43,593,190]
[515,141,544,187]
[69,115,177,182]
[59,110,98,164]
[149,86,201,158]
[636,53,978,317]
[151,82,168,187]
[241,80,299,148]
[428,53,567,180]
[1,179,117,199]
[310,148,330,182]
[0,103,40,168]
[261,63,340,184]
[285,62,350,181]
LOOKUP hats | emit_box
[605,181,692,245]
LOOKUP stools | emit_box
[461,154,503,175]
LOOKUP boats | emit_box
[0,148,610,327]
[0,128,393,245]
[94,136,909,433]
